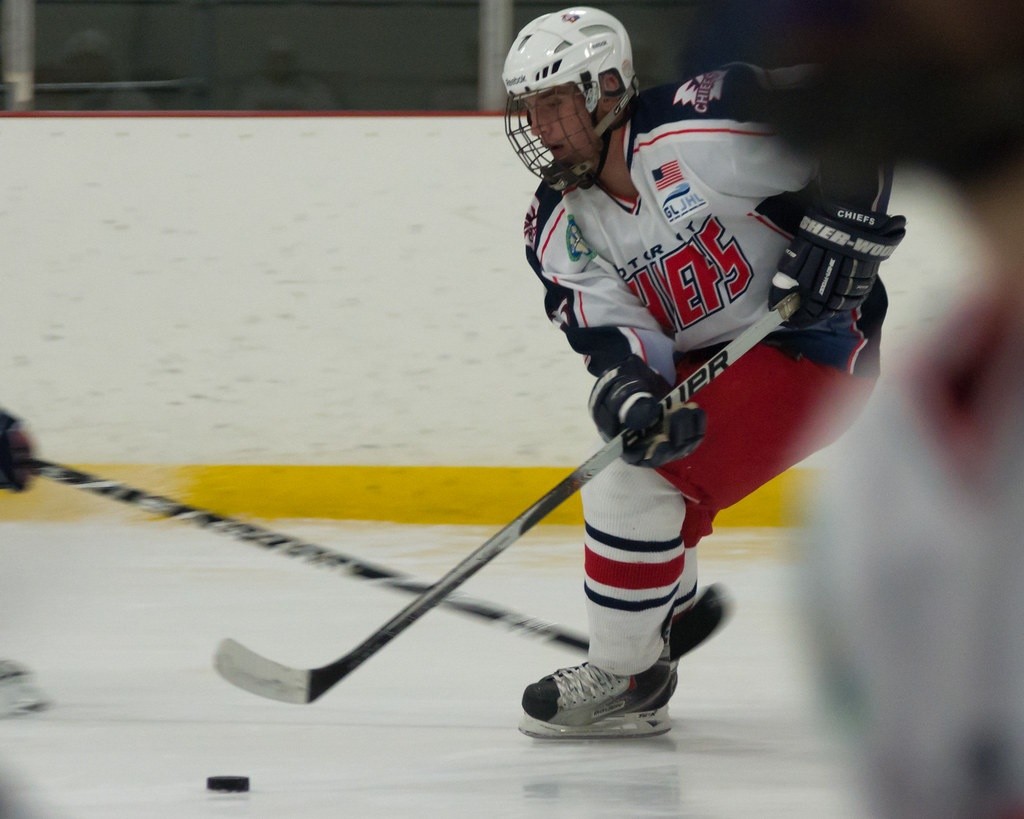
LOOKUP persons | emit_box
[499,4,906,742]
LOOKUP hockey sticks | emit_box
[212,287,796,705]
[15,452,725,672]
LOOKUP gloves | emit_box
[586,352,707,468]
[766,193,907,337]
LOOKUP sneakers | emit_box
[515,586,726,742]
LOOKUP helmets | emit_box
[502,6,638,115]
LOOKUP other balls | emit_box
[206,775,249,794]
